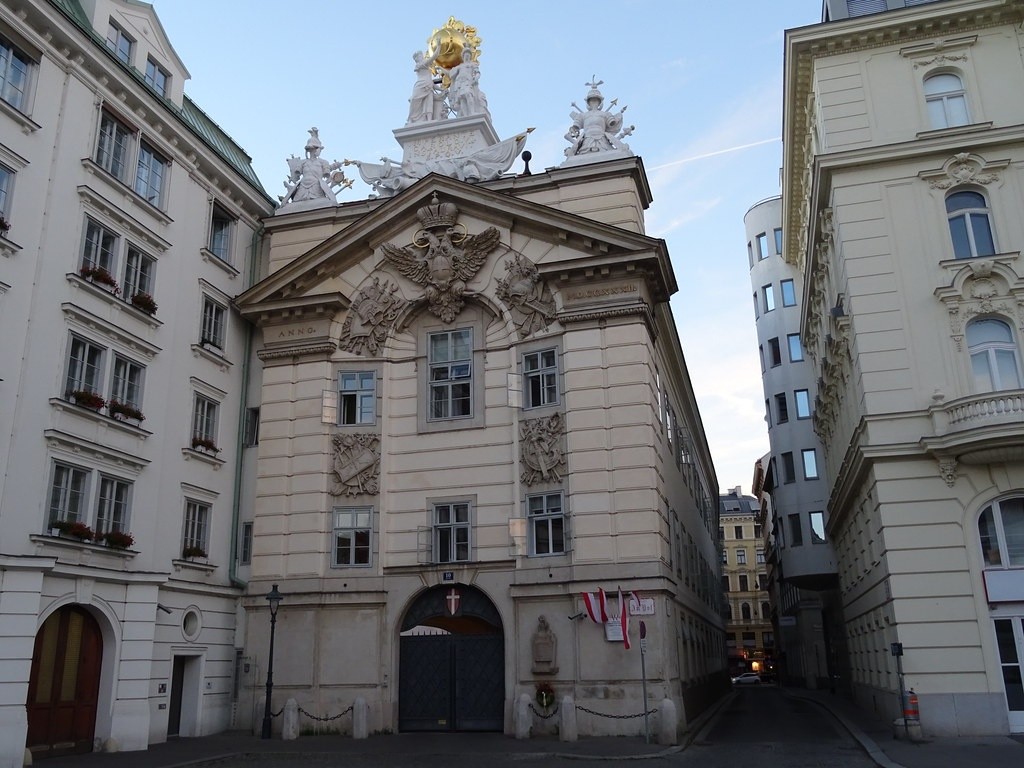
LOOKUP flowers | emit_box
[103,531,136,549]
[52,520,96,541]
[132,294,157,315]
[65,389,109,410]
[191,437,222,454]
[110,399,146,422]
[80,266,121,295]
[184,547,209,558]
[199,337,222,350]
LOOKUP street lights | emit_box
[261,583,283,740]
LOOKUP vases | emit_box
[70,399,95,412]
[188,557,208,564]
[133,303,151,316]
[115,414,142,427]
[195,444,218,458]
[85,274,117,295]
[52,528,82,542]
[206,344,225,358]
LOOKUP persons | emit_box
[281,126,337,207]
[449,47,481,116]
[940,462,958,480]
[563,74,630,156]
[406,37,442,123]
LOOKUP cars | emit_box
[732,672,761,685]
